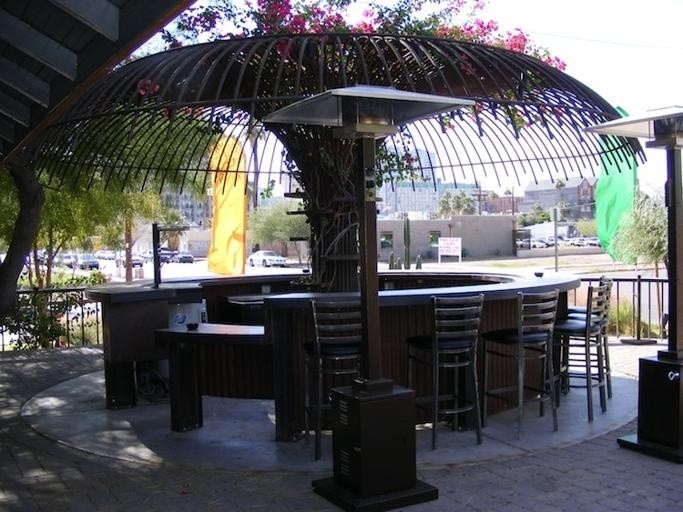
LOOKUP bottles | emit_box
[173,303,188,328]
[199,298,208,324]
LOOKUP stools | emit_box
[538,285,608,421]
[481,289,560,442]
[567,276,611,333]
[302,298,364,462]
[407,293,486,450]
[561,281,614,400]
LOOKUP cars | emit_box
[37,250,115,270]
[248,250,286,267]
[142,248,194,263]
[516,235,601,249]
[123,255,143,267]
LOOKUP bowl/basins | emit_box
[185,322,200,331]
